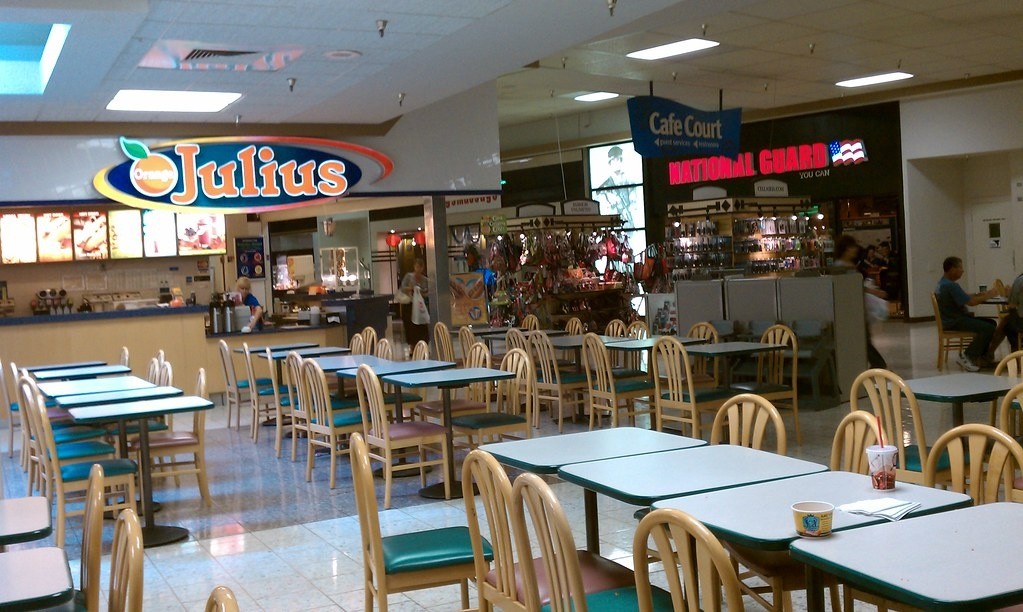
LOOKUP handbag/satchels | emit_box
[411,286,430,325]
[395,287,413,304]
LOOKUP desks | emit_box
[0,295,1023,612]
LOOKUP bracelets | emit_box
[976,292,978,296]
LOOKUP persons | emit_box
[981,273,1023,368]
[934,257,1001,372]
[400,258,429,357]
[237,277,262,330]
[833,235,901,369]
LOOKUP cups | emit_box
[979,286,987,292]
[866,445,898,491]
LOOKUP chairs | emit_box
[844,424,1022,612]
[0,314,801,612]
[722,409,890,609]
[848,368,971,496]
[992,279,1012,323]
[973,349,1022,506]
[929,291,976,372]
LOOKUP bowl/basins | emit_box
[791,501,835,539]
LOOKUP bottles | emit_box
[165,281,170,293]
[82,301,89,313]
[160,281,165,293]
[191,291,196,304]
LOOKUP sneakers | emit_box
[955,348,980,372]
